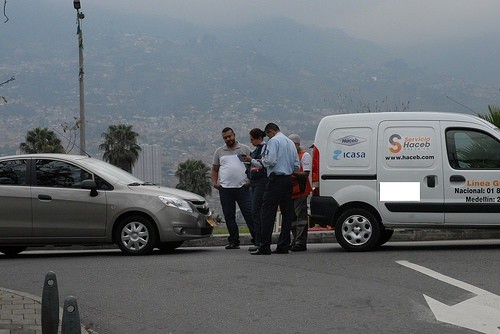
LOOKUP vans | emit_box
[308,111,500,253]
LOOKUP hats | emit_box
[287,133,301,144]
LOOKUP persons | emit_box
[211,127,253,249]
[251,123,297,255]
[243,128,268,251]
[288,133,312,252]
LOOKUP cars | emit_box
[0,152,219,257]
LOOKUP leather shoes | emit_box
[248,246,261,251]
[250,249,271,256]
[225,243,240,249]
[271,248,289,255]
[288,244,307,252]
